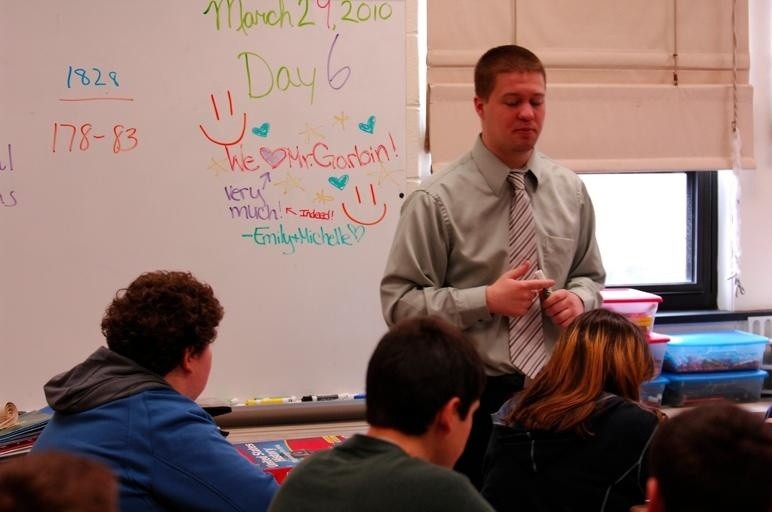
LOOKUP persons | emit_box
[265,316,496,511]
[637,402,772,511]
[378,42,607,490]
[32,269,281,511]
[482,306,668,512]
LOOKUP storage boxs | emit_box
[597,284,769,408]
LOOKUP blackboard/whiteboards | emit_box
[0,0,421,426]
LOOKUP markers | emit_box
[247,394,365,406]
[535,269,551,298]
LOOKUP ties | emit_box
[507,167,547,381]
